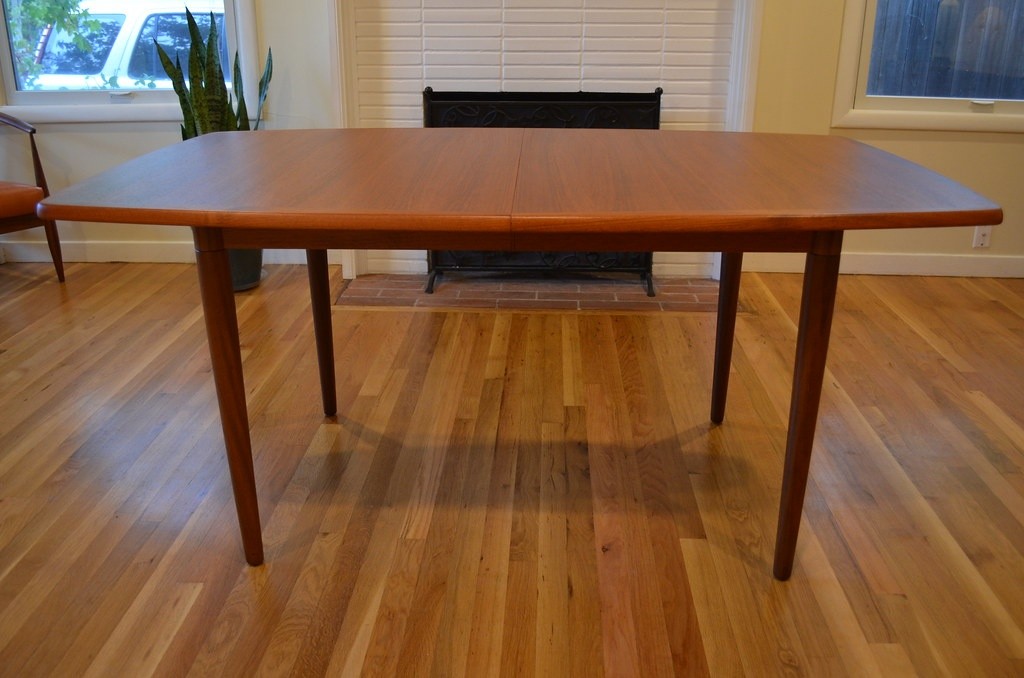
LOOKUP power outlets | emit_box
[972,225,992,248]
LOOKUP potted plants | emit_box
[154,7,273,292]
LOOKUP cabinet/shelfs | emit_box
[423,86,663,295]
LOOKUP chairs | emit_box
[0,112,66,284]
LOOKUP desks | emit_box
[35,127,1004,580]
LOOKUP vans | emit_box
[19,0,234,93]
